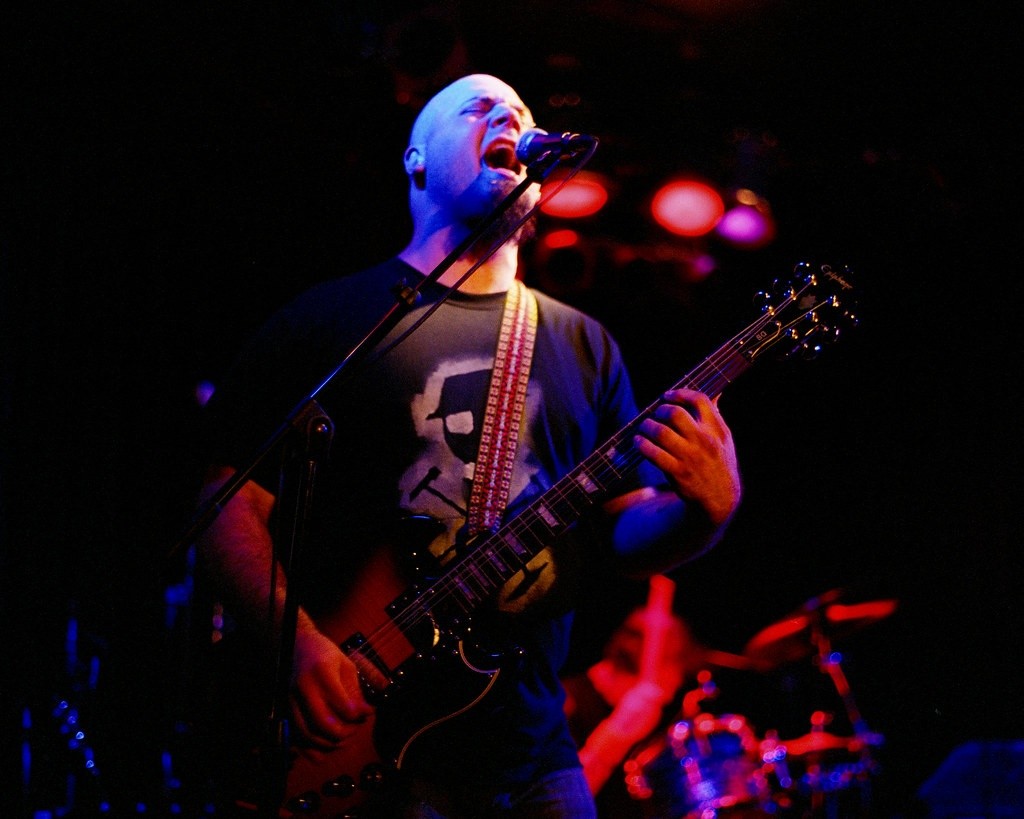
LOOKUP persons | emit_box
[197,69,745,819]
[559,598,882,819]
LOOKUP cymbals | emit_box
[745,600,894,664]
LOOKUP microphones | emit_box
[515,127,599,166]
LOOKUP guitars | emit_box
[205,258,865,819]
